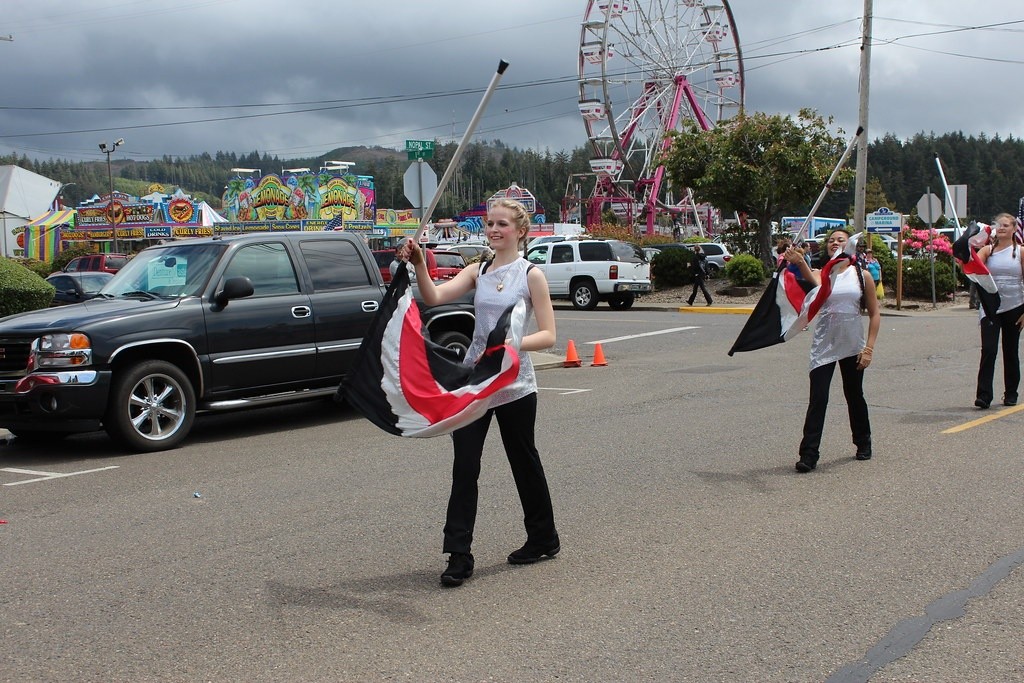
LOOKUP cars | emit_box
[882,239,913,271]
[44,271,134,307]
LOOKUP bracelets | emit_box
[862,346,874,356]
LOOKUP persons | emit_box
[402,199,561,585]
[856,240,883,312]
[784,230,880,472]
[685,245,713,307]
[771,237,811,331]
[974,213,1024,409]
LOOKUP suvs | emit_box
[522,237,653,312]
[62,253,129,275]
[372,247,466,287]
[641,243,733,279]
[435,242,494,260]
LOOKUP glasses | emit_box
[859,246,865,248]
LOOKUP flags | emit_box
[335,261,526,439]
[1015,203,1024,245]
[953,220,1002,320]
[730,232,861,352]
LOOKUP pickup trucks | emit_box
[0,230,475,452]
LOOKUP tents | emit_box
[24,210,110,265]
[198,200,229,226]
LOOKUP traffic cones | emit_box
[564,339,582,367]
[590,343,608,365]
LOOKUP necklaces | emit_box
[491,256,512,289]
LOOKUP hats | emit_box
[865,248,873,253]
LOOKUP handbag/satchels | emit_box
[876,280,884,300]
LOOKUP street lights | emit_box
[99,138,124,253]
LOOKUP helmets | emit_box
[858,240,866,246]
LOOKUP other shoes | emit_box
[975,398,990,408]
[796,455,817,471]
[1004,392,1017,406]
[686,300,692,306]
[507,536,561,564]
[856,448,872,460]
[440,553,474,586]
[801,326,808,331]
[706,300,713,306]
